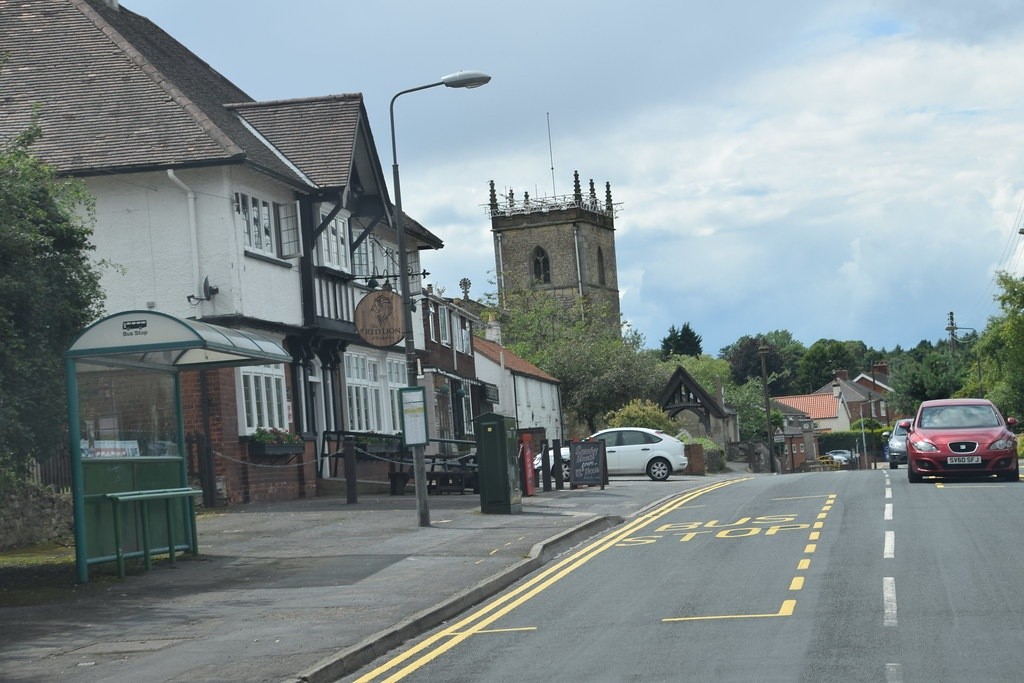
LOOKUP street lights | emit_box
[389,69,494,529]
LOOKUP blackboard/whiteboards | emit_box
[570,441,603,483]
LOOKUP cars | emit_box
[882,418,915,469]
[898,398,1020,484]
[819,449,860,466]
[532,426,689,481]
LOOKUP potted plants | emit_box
[249,424,305,457]
[357,428,401,456]
